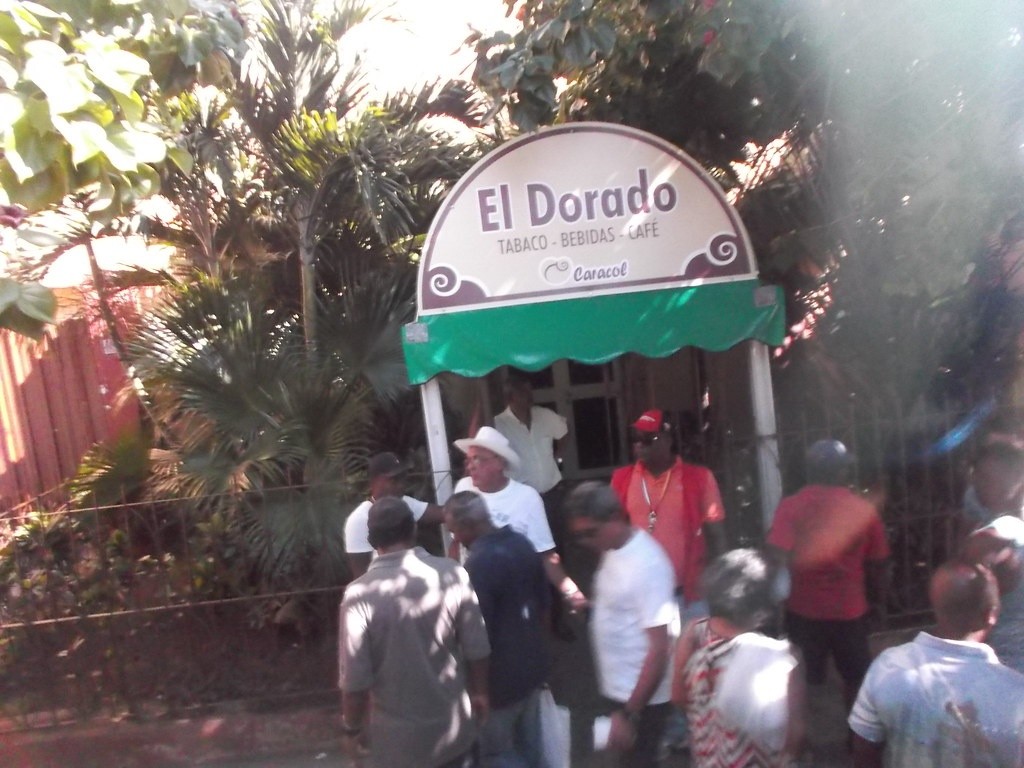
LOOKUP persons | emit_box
[338,363,1024,768]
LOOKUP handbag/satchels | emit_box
[708,629,795,750]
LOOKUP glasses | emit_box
[630,433,659,446]
[465,453,498,467]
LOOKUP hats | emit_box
[632,407,672,433]
[455,426,521,472]
[368,452,407,479]
[365,496,414,530]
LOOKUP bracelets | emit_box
[562,584,579,596]
[341,724,361,737]
[623,707,641,725]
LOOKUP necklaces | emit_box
[371,496,376,503]
[640,469,671,532]
